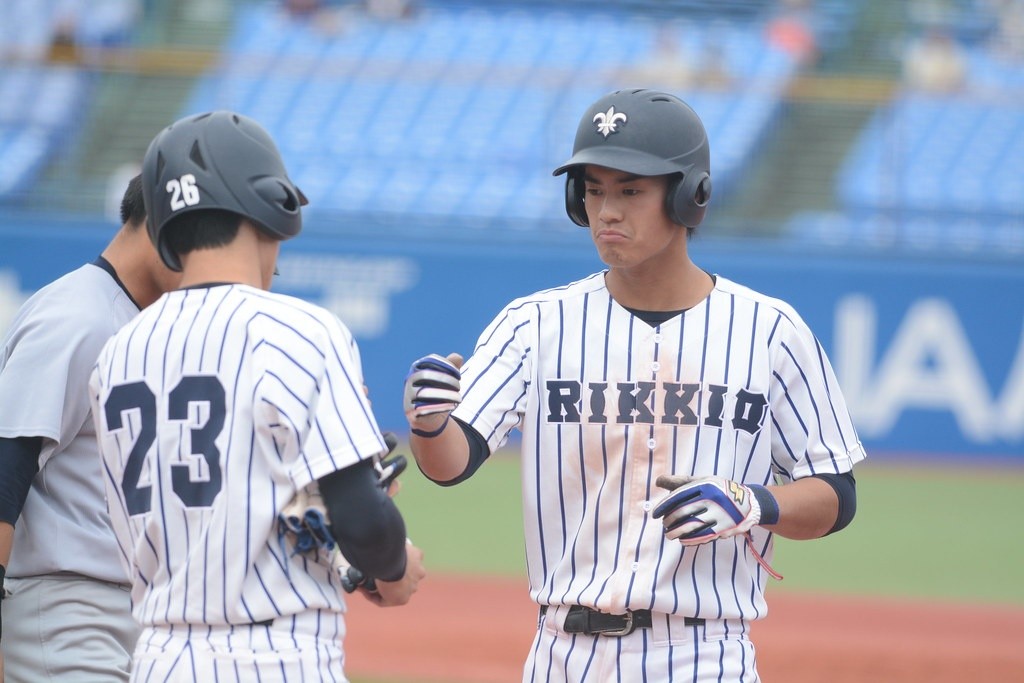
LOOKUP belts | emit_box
[540,604,706,637]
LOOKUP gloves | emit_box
[651,473,779,547]
[336,563,378,598]
[403,353,463,437]
[276,433,407,555]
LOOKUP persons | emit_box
[85,109,426,682]
[0,171,190,683]
[402,86,869,682]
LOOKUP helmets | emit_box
[553,87,711,229]
[141,111,309,271]
